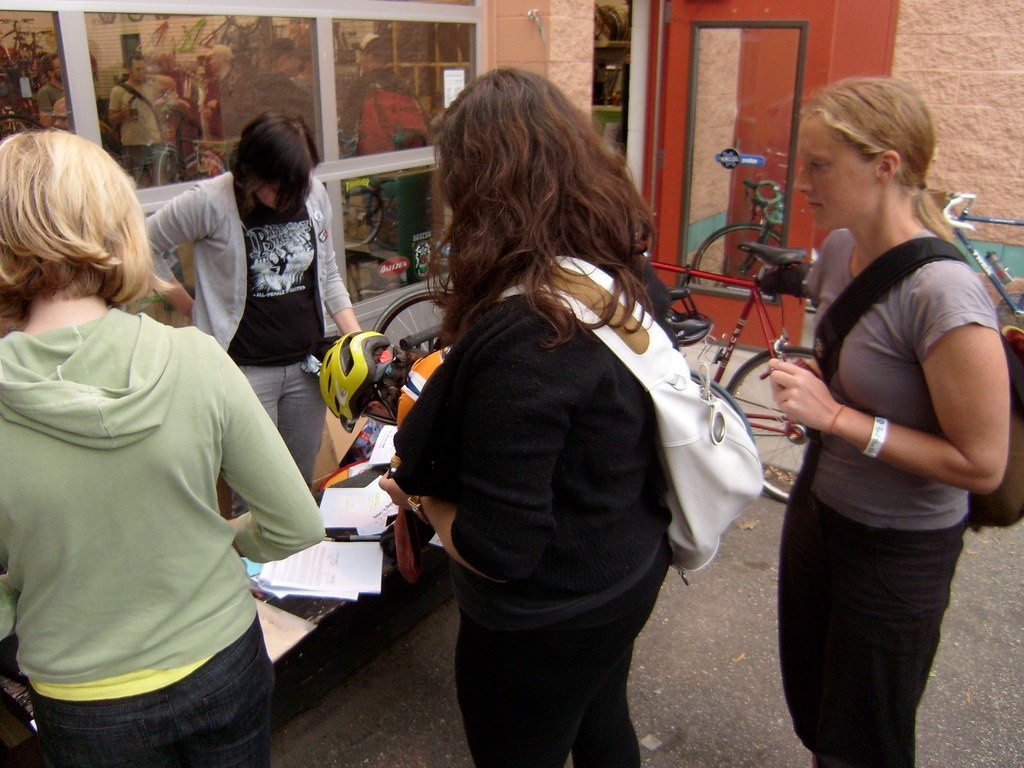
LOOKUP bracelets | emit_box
[862,416,887,458]
[827,404,846,435]
[407,496,430,524]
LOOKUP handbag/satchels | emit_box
[970,309,1024,536]
[659,374,765,589]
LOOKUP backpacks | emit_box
[356,69,432,156]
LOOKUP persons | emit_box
[767,76,1010,768]
[144,110,362,520]
[379,69,674,768]
[0,131,326,768]
[38,52,70,131]
[108,37,317,190]
[338,36,428,158]
[319,330,452,431]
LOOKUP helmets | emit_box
[318,328,390,434]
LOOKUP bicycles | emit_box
[688,177,788,300]
[940,189,1024,330]
[372,212,828,507]
[0,115,399,250]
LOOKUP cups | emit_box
[129,108,138,121]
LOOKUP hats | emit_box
[205,44,235,62]
[269,37,305,55]
[155,51,173,61]
[351,34,389,58]
[50,96,67,117]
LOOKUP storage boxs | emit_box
[216,405,454,731]
